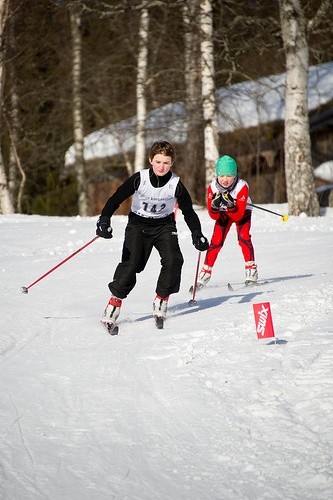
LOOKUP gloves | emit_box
[192,233,210,251]
[222,190,235,209]
[211,193,222,210]
[96,222,113,238]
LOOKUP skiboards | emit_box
[100,314,164,335]
[189,277,282,293]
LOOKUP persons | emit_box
[96,140,210,331]
[190,155,258,287]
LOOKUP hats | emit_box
[217,155,237,177]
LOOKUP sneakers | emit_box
[196,265,212,289]
[101,295,122,324]
[244,261,258,284]
[153,295,168,318]
